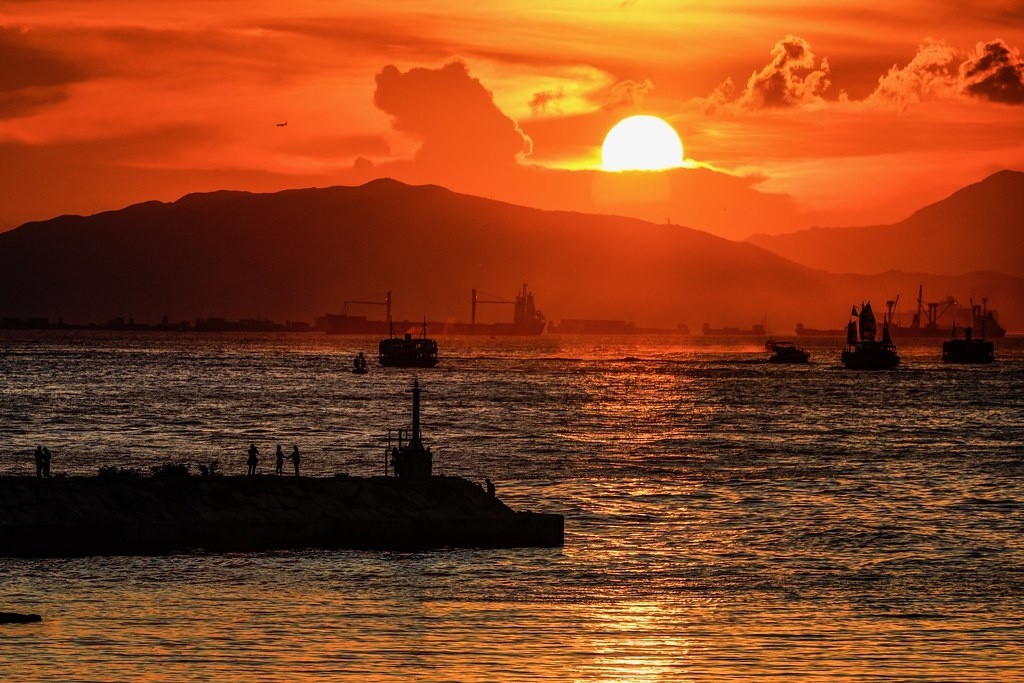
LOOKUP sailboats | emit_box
[841,294,900,371]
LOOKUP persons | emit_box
[276,445,300,476]
[247,443,259,475]
[34,445,52,477]
[486,478,495,496]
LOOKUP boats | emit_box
[377,315,440,369]
[941,317,995,365]
[766,340,811,365]
